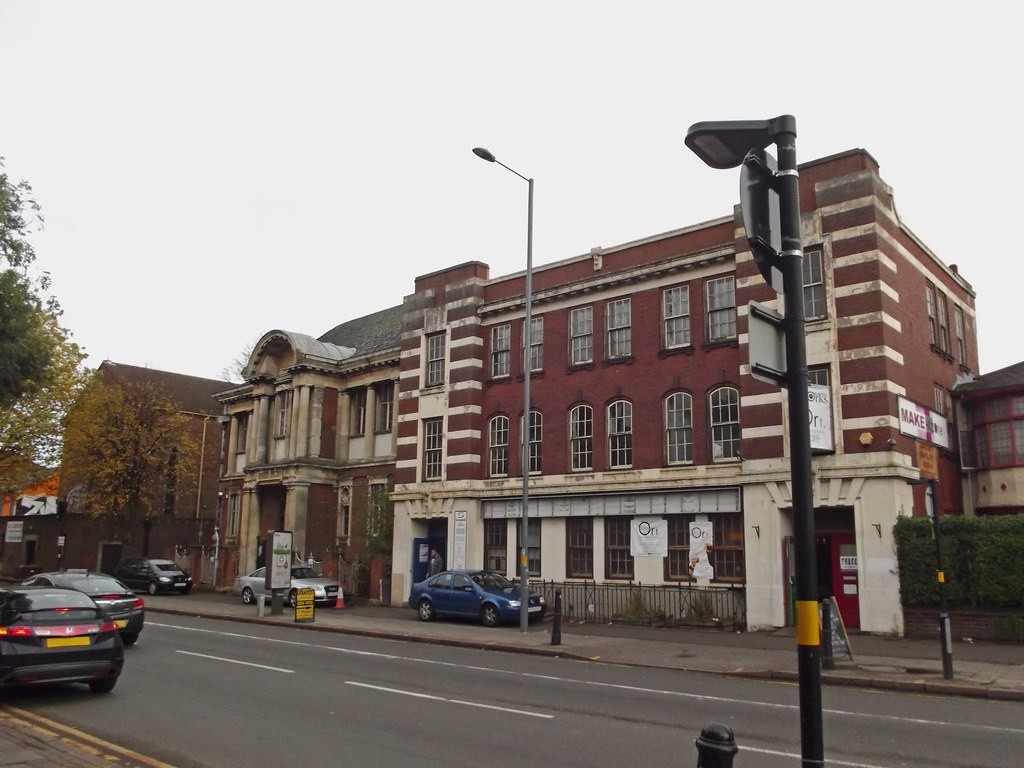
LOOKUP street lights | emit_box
[472,147,534,632]
[683,115,825,768]
[907,478,954,680]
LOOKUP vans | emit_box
[115,556,193,595]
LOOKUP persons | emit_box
[426,548,443,579]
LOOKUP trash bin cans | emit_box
[378,571,391,607]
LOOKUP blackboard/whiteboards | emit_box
[817,596,852,660]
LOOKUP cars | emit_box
[21,568,144,644]
[0,585,125,691]
[232,565,338,607]
[410,569,546,626]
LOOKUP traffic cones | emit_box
[334,580,346,609]
